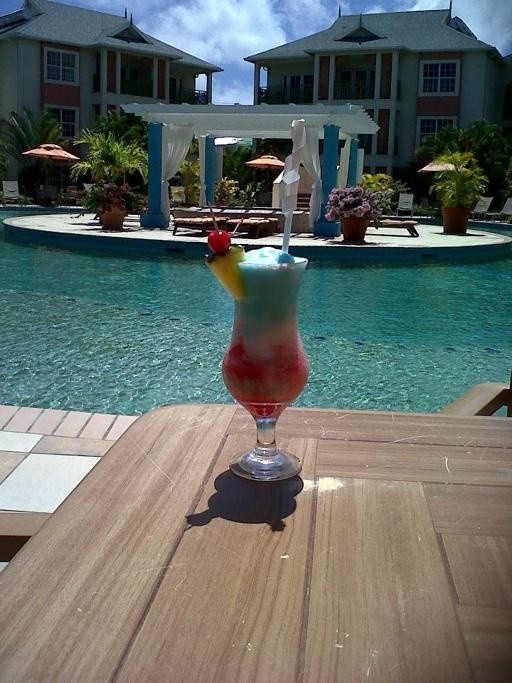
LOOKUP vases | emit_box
[340,219,368,242]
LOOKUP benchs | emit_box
[370,219,419,237]
[173,216,279,236]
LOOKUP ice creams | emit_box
[205,230,311,414]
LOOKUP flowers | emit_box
[325,184,383,229]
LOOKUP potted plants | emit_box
[70,111,149,229]
[428,146,489,234]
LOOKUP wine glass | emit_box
[220,248,312,482]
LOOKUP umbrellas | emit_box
[417,160,472,175]
[245,154,286,191]
[21,143,81,187]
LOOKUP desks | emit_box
[0,404,511,682]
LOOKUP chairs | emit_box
[39,185,78,207]
[3,181,19,201]
